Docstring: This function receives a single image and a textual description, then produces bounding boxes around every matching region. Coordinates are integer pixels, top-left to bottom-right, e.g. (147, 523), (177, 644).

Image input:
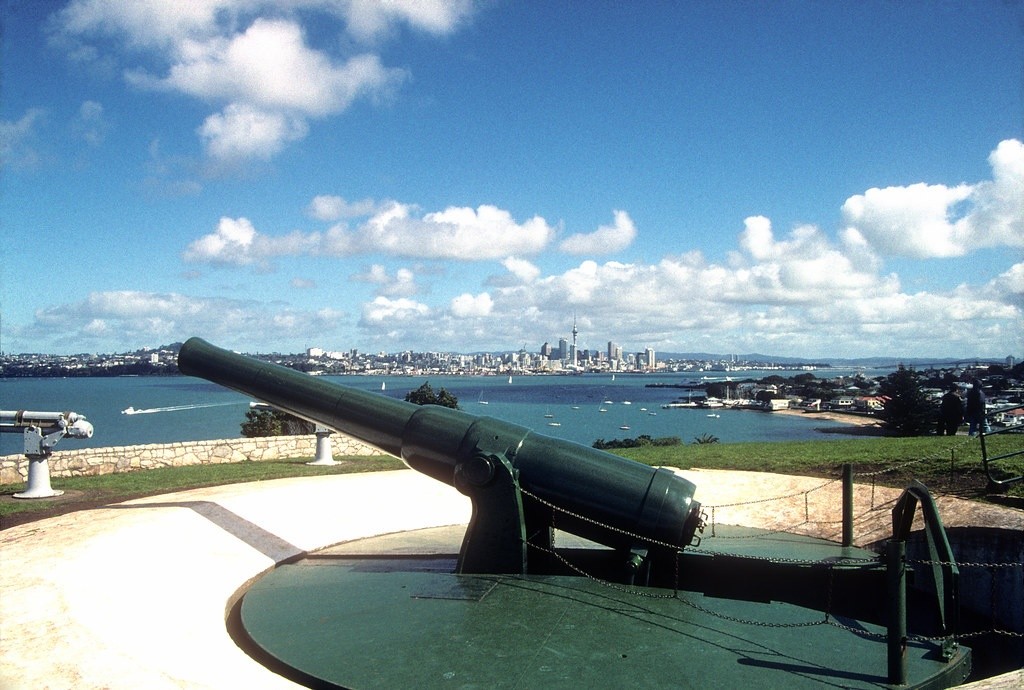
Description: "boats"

(693, 397), (724, 408)
(805, 398), (826, 413)
(120, 407), (135, 415)
